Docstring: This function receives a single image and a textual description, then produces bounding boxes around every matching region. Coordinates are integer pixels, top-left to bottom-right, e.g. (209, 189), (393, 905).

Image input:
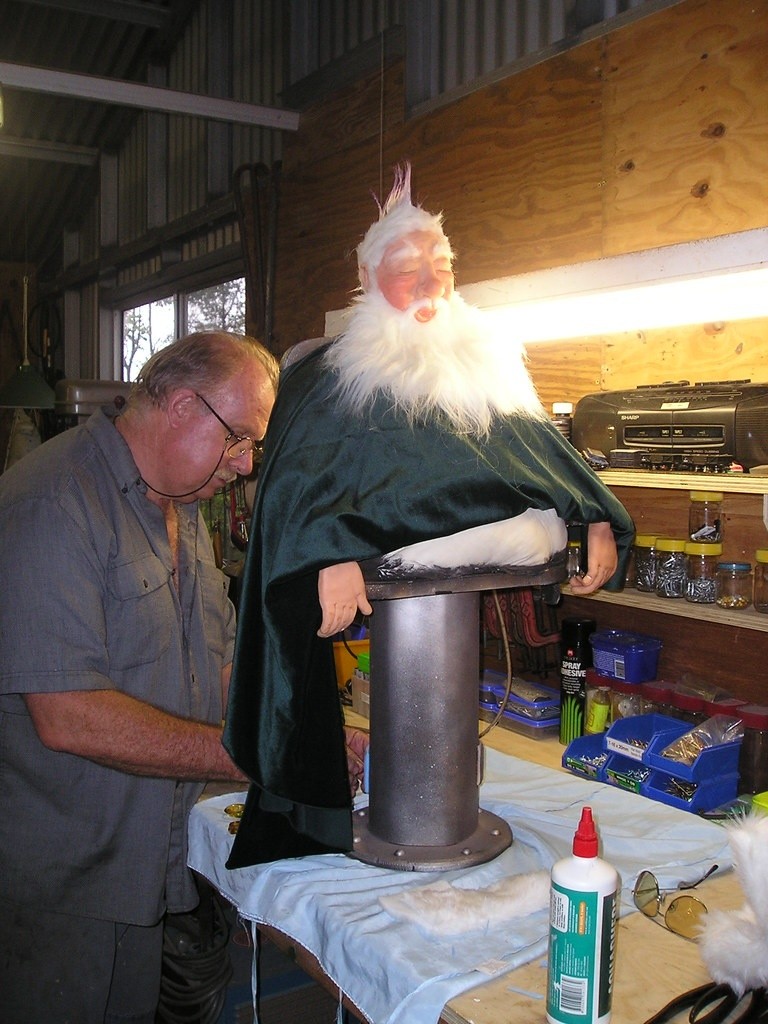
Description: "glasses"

(630, 865), (719, 945)
(195, 393), (264, 463)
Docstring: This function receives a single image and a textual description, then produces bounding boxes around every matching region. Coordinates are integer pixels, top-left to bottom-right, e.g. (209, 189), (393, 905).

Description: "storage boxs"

(333, 628), (743, 815)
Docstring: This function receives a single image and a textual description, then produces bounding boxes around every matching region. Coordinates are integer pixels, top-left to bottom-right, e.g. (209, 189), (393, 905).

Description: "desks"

(186, 687), (768, 1024)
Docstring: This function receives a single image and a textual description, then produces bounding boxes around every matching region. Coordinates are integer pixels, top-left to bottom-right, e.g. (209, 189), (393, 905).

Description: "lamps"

(0, 276), (57, 411)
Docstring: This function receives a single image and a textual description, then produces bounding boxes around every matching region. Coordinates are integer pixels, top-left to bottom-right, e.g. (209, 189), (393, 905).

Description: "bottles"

(559, 621), (767, 795)
(558, 490), (768, 615)
(548, 402), (573, 446)
(546, 806), (618, 1023)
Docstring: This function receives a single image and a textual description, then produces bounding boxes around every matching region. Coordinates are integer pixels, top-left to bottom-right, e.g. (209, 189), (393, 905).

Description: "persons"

(0, 330), (368, 1023)
(218, 160), (636, 868)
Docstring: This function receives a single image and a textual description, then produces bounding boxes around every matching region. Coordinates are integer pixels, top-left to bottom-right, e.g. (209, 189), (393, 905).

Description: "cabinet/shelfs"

(554, 467), (768, 633)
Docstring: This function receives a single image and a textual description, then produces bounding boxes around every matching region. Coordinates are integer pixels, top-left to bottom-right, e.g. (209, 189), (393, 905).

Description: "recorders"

(572, 378), (768, 473)
